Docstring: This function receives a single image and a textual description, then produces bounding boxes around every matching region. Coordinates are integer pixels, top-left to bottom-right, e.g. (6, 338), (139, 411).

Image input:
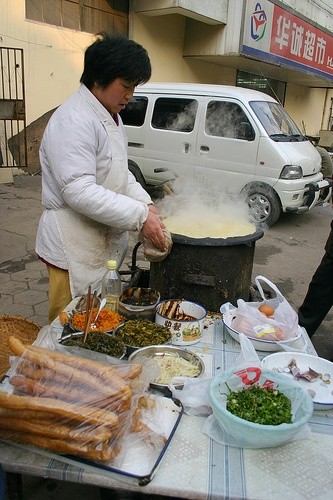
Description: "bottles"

(101, 259), (122, 314)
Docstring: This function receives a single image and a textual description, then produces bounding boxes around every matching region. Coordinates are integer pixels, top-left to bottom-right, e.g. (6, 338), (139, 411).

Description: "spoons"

(90, 299), (107, 330)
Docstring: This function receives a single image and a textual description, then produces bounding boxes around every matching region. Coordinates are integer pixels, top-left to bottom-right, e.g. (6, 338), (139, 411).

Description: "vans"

(118, 81), (333, 230)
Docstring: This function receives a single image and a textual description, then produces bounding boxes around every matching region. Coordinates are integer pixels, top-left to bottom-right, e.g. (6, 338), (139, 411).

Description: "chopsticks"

(81, 286), (97, 343)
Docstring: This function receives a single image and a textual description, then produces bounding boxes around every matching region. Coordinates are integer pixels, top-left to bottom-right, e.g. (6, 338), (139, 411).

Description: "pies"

(161, 215), (257, 239)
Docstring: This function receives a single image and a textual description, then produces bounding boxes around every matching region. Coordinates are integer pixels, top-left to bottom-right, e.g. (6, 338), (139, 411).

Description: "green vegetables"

(117, 318), (171, 347)
(222, 382), (293, 426)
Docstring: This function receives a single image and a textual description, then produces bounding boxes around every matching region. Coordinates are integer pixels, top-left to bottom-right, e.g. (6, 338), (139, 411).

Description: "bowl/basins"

(57, 331), (127, 360)
(117, 286), (160, 321)
(112, 322), (173, 357)
(155, 300), (207, 346)
(128, 345), (206, 396)
(221, 308), (301, 352)
(261, 352), (333, 411)
(67, 309), (127, 333)
(208, 366), (314, 448)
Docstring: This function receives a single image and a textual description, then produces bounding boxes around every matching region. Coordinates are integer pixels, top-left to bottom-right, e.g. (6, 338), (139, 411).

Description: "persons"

(312, 140), (333, 201)
(34, 31), (165, 326)
(298, 220), (333, 338)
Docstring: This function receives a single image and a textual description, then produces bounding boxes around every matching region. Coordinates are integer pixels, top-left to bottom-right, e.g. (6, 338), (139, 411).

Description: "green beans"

(58, 330), (127, 358)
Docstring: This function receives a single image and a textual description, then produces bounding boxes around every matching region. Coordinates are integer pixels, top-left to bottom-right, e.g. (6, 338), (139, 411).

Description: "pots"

(160, 210), (264, 245)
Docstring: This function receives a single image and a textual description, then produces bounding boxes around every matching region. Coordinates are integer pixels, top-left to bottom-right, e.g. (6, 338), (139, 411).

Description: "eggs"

(238, 305), (283, 341)
